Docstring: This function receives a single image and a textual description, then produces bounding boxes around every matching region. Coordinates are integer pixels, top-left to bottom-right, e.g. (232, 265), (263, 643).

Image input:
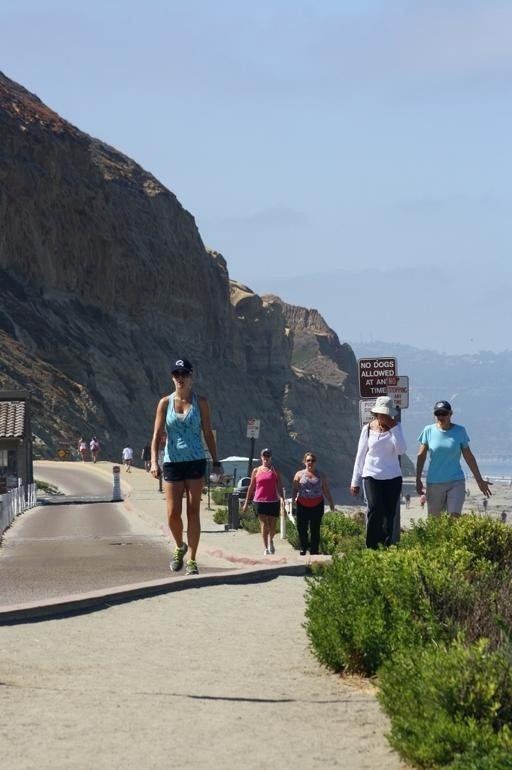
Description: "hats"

(261, 448), (272, 456)
(171, 359), (192, 373)
(434, 401), (451, 413)
(370, 396), (399, 416)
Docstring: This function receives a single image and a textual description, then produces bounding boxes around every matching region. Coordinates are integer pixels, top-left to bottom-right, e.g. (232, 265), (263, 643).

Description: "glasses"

(435, 411), (448, 416)
(174, 371), (188, 378)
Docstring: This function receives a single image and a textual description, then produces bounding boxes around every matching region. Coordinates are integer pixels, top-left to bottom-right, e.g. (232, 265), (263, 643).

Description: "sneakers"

(170, 543), (187, 571)
(185, 560), (199, 575)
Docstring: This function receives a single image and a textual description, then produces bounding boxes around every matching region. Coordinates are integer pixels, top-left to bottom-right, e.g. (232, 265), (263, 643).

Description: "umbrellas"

(210, 455), (259, 486)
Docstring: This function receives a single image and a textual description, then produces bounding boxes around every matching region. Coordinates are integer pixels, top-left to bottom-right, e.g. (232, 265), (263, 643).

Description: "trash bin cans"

(233, 477), (255, 529)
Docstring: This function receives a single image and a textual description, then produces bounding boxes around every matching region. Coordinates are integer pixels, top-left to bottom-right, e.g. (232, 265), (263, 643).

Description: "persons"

(420, 492), (425, 508)
(416, 401), (494, 520)
(481, 498), (488, 512)
(292, 453), (336, 555)
(244, 448), (286, 555)
(349, 396), (407, 551)
(122, 444), (133, 473)
(466, 488), (469, 497)
(90, 435), (101, 463)
(151, 358), (223, 577)
(141, 442), (150, 472)
(404, 493), (410, 507)
(79, 436), (88, 462)
(500, 511), (507, 522)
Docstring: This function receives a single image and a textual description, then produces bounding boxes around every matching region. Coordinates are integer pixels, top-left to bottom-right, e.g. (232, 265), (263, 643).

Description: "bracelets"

(246, 498), (250, 500)
(212, 462), (221, 467)
